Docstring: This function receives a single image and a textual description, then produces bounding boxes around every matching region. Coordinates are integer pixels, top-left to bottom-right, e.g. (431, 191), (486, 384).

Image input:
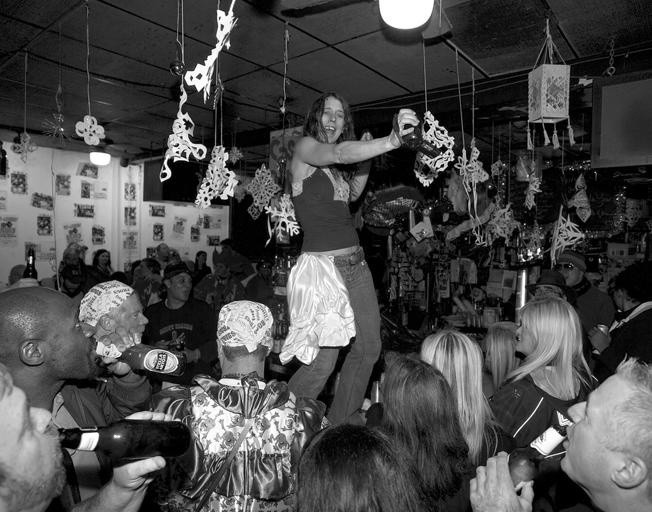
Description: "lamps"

(376, 1), (435, 31)
(87, 142), (113, 168)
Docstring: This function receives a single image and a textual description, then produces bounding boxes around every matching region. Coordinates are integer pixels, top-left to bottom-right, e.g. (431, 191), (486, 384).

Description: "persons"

(278, 91), (420, 427)
(0, 241), (652, 512)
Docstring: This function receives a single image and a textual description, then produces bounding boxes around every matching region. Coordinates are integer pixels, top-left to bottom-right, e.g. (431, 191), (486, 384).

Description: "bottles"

(24, 247), (38, 277)
(402, 127), (421, 149)
(56, 418), (192, 459)
(270, 254), (299, 361)
(91, 339), (187, 378)
(508, 423), (565, 496)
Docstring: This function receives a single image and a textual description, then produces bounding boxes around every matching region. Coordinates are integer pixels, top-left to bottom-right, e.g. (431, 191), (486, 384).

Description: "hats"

(524, 271), (570, 297)
(257, 258), (274, 272)
(160, 261), (192, 290)
(557, 249), (588, 273)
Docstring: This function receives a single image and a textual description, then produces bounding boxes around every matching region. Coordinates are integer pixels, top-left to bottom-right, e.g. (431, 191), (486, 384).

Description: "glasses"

(554, 264), (574, 271)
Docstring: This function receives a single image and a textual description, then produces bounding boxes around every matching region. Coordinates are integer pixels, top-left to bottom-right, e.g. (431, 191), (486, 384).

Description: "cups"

(371, 381), (384, 404)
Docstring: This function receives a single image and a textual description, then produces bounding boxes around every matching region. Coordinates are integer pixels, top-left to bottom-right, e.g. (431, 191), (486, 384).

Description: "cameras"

(402, 114), (440, 159)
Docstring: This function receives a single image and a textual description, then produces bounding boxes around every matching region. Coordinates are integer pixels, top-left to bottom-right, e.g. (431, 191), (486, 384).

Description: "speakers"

(592, 70), (652, 168)
(229, 193), (268, 263)
(143, 160), (230, 206)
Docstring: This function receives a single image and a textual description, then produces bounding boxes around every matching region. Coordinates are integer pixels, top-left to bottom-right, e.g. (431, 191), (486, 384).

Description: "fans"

(278, 1), (468, 41)
